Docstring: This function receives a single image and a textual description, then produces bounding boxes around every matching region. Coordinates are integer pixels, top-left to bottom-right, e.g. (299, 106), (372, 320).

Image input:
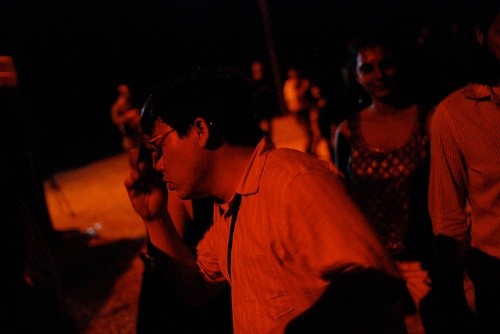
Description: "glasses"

(141, 118), (213, 156)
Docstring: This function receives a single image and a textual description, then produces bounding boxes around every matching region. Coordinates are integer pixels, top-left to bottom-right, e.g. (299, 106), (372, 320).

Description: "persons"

(334, 32), (474, 334)
(425, 0), (500, 334)
(110, 60), (351, 175)
(123, 66), (419, 334)
